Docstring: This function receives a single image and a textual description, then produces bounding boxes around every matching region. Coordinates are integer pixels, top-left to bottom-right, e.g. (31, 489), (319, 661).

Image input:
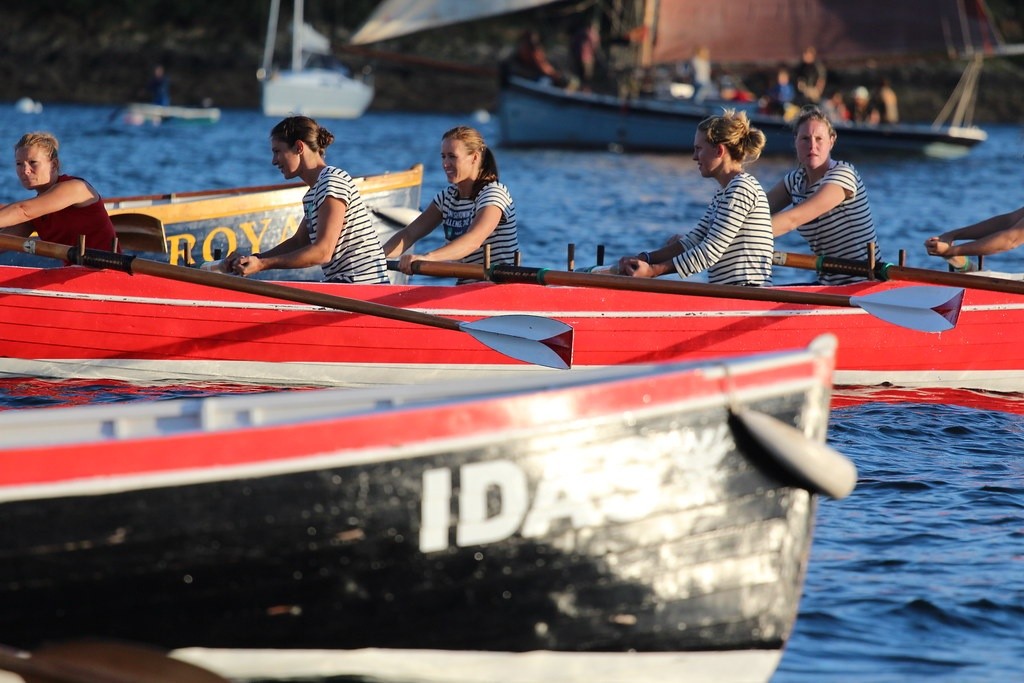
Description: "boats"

(103, 164), (427, 284)
(0, 262), (1023, 414)
(0, 332), (859, 683)
(111, 102), (220, 128)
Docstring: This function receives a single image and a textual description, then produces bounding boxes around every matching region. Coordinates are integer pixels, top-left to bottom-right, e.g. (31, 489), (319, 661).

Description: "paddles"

(386, 256), (967, 333)
(0, 227), (575, 371)
(770, 247), (1024, 298)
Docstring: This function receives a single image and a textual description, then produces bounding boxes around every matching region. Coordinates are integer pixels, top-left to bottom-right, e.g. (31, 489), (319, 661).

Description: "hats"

(849, 85), (869, 99)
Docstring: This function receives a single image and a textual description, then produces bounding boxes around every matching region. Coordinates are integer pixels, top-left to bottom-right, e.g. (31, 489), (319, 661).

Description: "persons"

(767, 109), (884, 284)
(382, 125), (519, 284)
(0, 132), (125, 267)
(925, 206), (1024, 259)
(529, 37), (905, 120)
(619, 110), (772, 286)
(233, 115), (389, 284)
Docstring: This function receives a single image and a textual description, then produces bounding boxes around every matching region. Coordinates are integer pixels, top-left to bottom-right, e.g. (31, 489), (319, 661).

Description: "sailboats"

(493, 0), (991, 161)
(253, 0), (376, 118)
(330, 1), (560, 113)
